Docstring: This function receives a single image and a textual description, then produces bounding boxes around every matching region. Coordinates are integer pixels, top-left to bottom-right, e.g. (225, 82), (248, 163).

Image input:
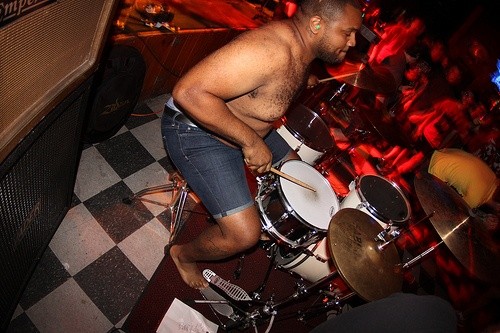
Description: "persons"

(302, 0), (500, 333)
(161, 0), (366, 289)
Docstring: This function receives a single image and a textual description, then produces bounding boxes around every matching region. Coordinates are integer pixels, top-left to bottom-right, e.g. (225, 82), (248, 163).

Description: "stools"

(121, 164), (201, 257)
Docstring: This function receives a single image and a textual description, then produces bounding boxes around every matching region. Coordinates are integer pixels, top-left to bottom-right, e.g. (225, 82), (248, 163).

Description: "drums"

(277, 100), (333, 166)
(276, 228), (338, 281)
(334, 172), (412, 238)
(255, 158), (340, 248)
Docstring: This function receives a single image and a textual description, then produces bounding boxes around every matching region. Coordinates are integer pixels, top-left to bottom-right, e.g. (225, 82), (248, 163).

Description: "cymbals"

(328, 207), (406, 302)
(360, 85), (415, 147)
(412, 169), (500, 287)
(324, 52), (399, 93)
